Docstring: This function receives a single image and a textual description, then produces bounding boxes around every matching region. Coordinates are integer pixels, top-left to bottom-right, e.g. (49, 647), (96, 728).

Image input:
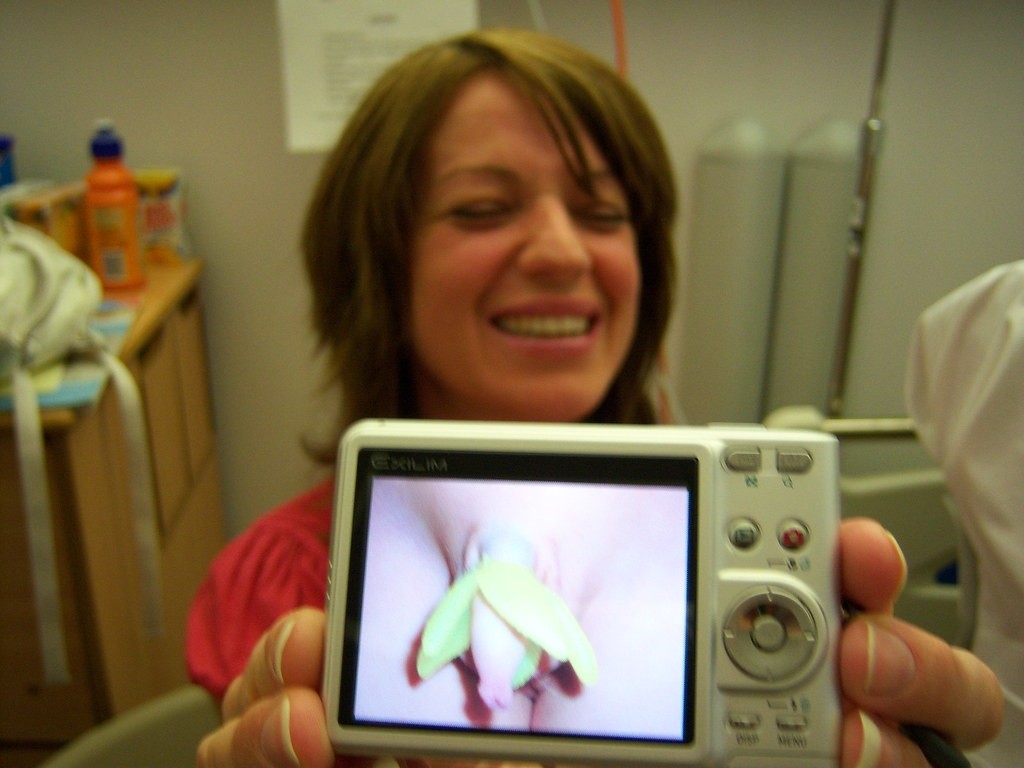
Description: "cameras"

(321, 419), (840, 768)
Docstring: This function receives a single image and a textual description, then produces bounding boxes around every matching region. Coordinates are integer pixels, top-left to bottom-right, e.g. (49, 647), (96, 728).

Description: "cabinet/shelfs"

(1, 257), (226, 768)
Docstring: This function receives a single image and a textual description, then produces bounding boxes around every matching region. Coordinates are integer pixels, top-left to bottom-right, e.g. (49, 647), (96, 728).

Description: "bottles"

(87, 124), (145, 291)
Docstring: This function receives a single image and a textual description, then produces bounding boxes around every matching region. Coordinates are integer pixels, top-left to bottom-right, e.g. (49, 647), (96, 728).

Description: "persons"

(182, 28), (1007, 768)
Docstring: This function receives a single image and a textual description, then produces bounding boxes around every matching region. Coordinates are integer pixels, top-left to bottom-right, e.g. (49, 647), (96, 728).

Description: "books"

(0, 299), (134, 410)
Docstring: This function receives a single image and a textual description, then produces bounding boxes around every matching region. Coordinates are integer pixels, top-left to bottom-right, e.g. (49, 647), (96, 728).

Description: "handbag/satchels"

(0, 217), (102, 373)
